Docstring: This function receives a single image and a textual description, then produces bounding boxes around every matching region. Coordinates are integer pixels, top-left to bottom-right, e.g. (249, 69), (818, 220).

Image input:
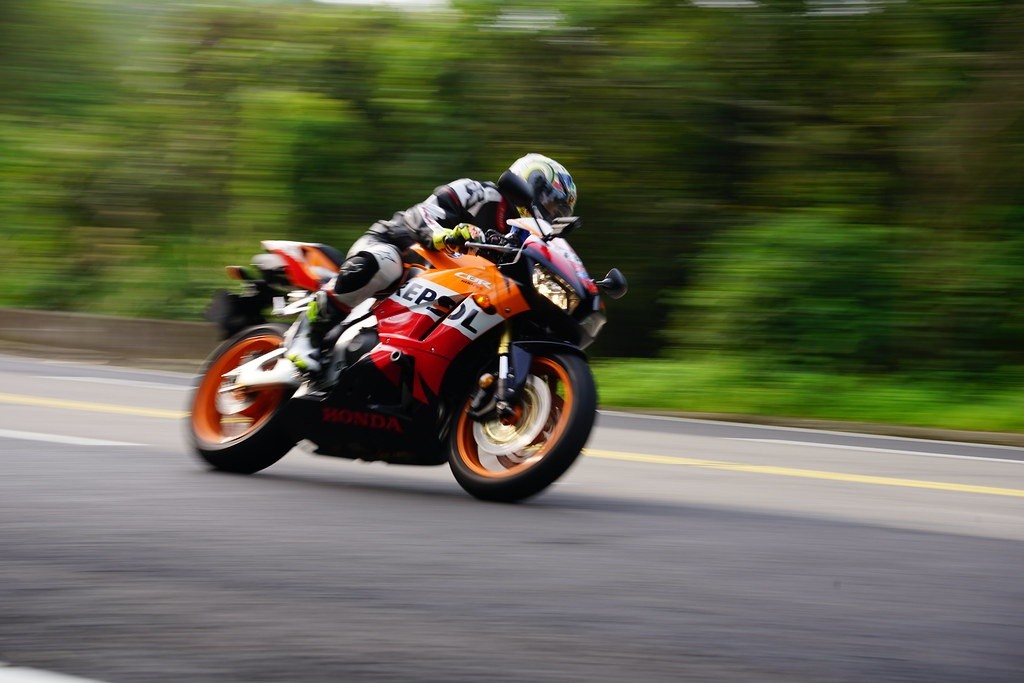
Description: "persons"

(283, 153), (577, 372)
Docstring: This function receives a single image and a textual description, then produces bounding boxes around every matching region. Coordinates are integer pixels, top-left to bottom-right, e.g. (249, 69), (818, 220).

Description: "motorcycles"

(188, 167), (628, 501)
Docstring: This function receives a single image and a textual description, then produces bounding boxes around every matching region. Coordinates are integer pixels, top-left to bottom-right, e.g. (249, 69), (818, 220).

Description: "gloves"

(434, 223), (486, 255)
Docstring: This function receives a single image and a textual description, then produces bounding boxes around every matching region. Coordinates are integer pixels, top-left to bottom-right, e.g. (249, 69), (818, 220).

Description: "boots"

(280, 289), (352, 371)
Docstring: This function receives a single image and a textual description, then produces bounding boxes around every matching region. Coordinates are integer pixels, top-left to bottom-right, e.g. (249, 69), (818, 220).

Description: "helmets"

(498, 152), (577, 223)
